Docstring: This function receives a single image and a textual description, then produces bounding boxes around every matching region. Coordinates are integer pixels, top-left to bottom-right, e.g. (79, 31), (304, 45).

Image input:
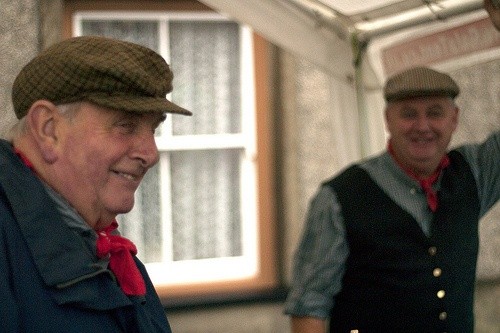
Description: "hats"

(382, 65), (460, 100)
(10, 34), (195, 117)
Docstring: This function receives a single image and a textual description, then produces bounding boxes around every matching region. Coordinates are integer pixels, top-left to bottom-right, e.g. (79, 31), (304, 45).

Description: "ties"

(387, 136), (449, 212)
(9, 144), (147, 296)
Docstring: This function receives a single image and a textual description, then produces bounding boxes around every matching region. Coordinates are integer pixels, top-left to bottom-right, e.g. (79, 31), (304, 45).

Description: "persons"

(283, 67), (500, 333)
(0, 37), (193, 333)
